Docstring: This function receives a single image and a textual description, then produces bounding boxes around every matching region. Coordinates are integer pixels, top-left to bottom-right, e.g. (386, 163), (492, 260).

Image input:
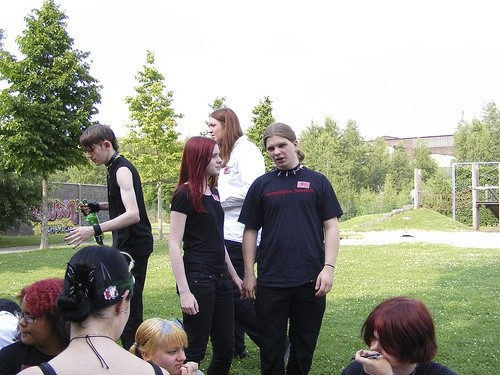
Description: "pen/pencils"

(352, 354), (382, 360)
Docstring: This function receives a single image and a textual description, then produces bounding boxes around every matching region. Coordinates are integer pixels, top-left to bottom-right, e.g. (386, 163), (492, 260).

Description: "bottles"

(82, 199), (104, 244)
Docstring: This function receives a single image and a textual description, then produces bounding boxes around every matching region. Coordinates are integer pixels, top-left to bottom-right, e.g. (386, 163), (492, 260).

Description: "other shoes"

(283, 335), (290, 362)
(233, 349), (247, 358)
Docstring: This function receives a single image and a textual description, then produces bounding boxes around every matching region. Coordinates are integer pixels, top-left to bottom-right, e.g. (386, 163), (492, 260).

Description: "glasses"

(141, 317), (184, 346)
(14, 308), (52, 323)
(120, 251), (135, 274)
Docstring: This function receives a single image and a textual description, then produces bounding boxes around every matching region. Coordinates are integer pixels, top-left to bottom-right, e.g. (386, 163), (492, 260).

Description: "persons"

(64, 124), (153, 350)
(340, 296), (457, 375)
(208, 108), (291, 363)
(168, 136), (256, 375)
(238, 123), (343, 375)
(0, 245), (205, 375)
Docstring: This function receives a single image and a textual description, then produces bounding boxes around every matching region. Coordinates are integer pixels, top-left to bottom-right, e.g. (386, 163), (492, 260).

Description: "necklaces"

(276, 163), (304, 177)
(105, 151), (120, 166)
(412, 363), (418, 375)
(69, 333), (116, 369)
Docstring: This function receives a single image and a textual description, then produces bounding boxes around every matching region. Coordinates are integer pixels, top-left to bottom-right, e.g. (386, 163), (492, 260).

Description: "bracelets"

(92, 223), (102, 237)
(324, 263), (335, 268)
